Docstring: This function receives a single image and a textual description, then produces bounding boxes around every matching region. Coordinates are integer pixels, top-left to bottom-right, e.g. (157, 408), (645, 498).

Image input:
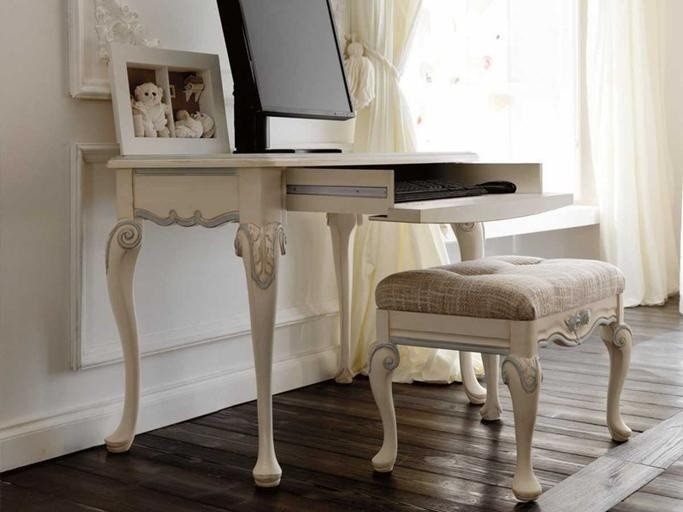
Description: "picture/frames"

(107, 41), (231, 157)
(66, 1), (234, 109)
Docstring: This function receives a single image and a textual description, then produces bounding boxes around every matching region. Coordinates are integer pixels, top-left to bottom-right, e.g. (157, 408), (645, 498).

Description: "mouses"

(479, 181), (516, 193)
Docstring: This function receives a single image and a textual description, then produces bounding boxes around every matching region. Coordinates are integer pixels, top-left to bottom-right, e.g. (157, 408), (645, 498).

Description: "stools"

(365, 254), (634, 500)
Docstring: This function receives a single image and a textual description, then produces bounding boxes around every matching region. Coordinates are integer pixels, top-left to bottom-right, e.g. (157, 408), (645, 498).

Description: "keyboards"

(395, 179), (488, 203)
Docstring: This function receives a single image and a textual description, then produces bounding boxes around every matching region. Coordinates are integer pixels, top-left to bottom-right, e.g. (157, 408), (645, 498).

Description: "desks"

(103, 150), (573, 486)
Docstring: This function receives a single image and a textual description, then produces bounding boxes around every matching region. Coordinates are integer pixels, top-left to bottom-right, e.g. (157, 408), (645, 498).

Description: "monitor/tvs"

(216, 0), (355, 153)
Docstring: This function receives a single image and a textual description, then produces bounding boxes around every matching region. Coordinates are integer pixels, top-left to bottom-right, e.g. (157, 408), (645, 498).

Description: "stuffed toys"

(134, 82), (170, 137)
(174, 110), (215, 138)
